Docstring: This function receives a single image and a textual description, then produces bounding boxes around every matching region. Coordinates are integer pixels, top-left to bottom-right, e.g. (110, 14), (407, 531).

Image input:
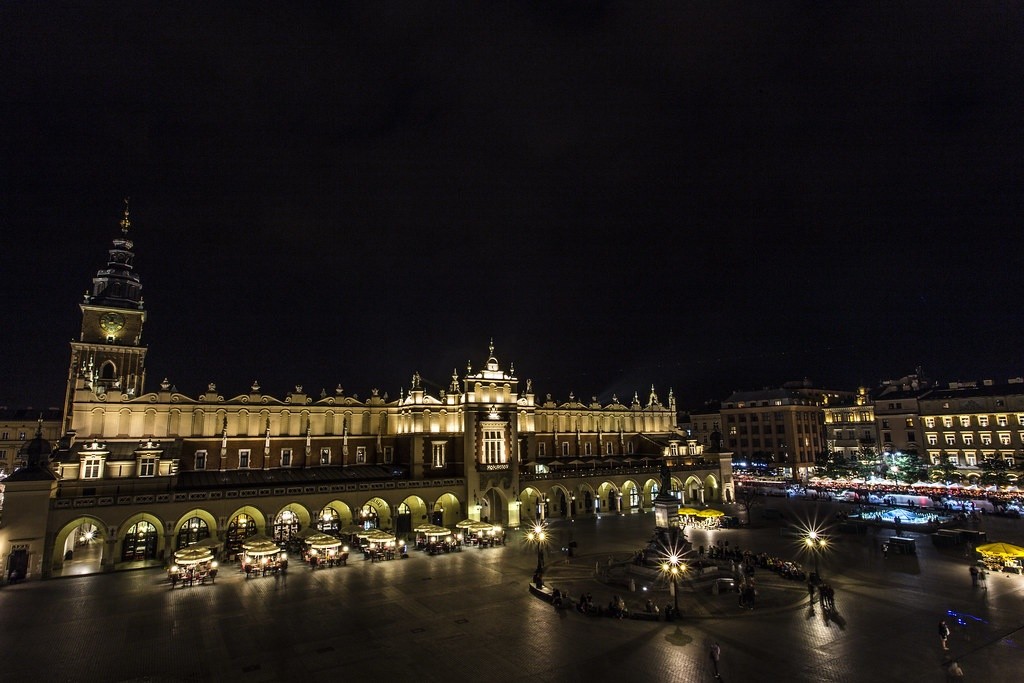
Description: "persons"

(709, 641), (721, 678)
(938, 618), (951, 651)
(969, 565), (989, 589)
(732, 563), (760, 613)
(775, 561), (821, 586)
(881, 542), (890, 561)
(948, 661), (963, 683)
(696, 539), (778, 565)
(646, 598), (684, 617)
(806, 582), (835, 609)
(907, 499), (915, 506)
(551, 588), (628, 622)
(894, 516), (902, 528)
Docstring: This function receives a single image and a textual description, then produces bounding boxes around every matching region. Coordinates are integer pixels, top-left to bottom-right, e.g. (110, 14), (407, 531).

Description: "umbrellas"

(174, 538), (225, 564)
(242, 533), (280, 563)
(294, 526), (342, 563)
(975, 543), (1024, 559)
(414, 523), (451, 541)
(677, 507), (725, 524)
(456, 518), (493, 537)
(339, 524), (396, 549)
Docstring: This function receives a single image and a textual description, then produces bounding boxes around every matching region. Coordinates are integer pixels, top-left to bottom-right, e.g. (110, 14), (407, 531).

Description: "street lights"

(806, 530), (828, 575)
(527, 524), (549, 566)
(661, 561), (686, 609)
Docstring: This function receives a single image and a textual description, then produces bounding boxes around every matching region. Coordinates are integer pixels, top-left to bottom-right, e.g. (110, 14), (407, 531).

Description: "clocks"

(99, 312), (125, 333)
